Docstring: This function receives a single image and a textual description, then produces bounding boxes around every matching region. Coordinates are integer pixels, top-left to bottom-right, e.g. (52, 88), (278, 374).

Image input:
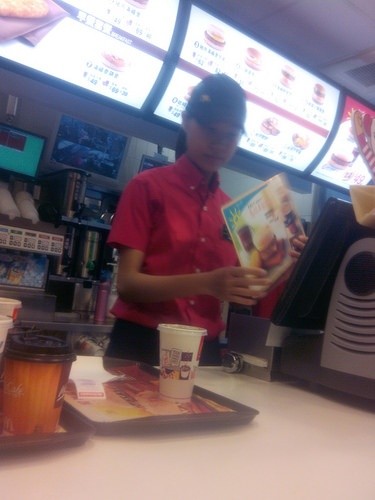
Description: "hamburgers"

(312, 83), (325, 105)
(245, 48), (261, 69)
(329, 148), (354, 169)
(280, 65), (296, 87)
(253, 225), (278, 261)
(203, 29), (225, 50)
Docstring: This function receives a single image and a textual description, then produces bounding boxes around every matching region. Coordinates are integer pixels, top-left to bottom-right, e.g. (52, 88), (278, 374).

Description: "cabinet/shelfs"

(42, 215), (113, 285)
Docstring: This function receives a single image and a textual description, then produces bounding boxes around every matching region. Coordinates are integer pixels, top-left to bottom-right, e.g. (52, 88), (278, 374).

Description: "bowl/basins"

(349, 184), (375, 227)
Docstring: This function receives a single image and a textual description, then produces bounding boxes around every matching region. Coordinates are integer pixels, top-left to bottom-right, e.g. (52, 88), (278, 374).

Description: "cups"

(236, 202), (298, 259)
(156, 323), (207, 398)
(0, 296), (77, 436)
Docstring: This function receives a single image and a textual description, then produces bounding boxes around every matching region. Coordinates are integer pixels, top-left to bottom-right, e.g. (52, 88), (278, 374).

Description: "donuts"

(103, 53), (127, 71)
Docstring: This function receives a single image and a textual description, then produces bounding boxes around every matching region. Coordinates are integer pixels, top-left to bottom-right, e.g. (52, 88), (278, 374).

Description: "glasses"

(197, 121), (244, 141)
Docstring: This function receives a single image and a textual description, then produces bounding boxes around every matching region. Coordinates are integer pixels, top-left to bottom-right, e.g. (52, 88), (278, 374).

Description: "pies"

(0, 0), (48, 18)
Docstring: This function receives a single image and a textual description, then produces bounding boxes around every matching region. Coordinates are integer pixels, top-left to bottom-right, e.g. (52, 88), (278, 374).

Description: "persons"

(107, 74), (312, 366)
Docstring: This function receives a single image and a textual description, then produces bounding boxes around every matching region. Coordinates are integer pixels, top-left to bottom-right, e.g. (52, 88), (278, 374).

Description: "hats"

(186, 74), (246, 121)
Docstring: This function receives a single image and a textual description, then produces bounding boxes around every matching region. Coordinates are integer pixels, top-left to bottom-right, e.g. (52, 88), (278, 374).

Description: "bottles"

(94, 278), (109, 324)
